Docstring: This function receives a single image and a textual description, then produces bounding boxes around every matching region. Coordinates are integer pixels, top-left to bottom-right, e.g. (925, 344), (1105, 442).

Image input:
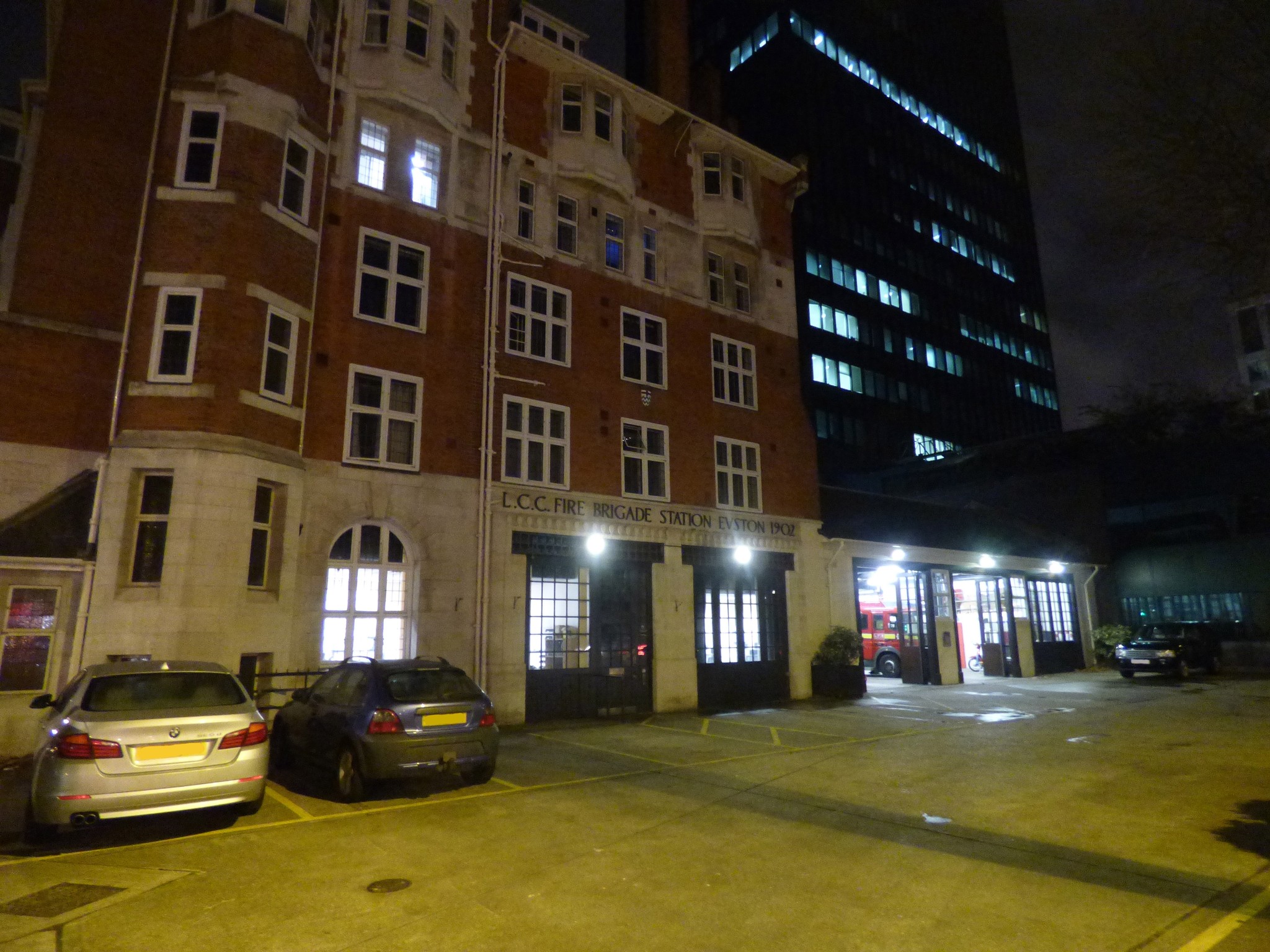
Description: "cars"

(30, 660), (270, 838)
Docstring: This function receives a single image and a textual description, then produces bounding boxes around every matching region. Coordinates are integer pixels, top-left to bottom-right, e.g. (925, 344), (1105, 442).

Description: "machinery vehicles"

(856, 599), (921, 680)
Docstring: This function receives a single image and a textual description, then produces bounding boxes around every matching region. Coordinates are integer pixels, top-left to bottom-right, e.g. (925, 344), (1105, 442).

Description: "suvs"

(1115, 620), (1212, 682)
(278, 652), (502, 797)
